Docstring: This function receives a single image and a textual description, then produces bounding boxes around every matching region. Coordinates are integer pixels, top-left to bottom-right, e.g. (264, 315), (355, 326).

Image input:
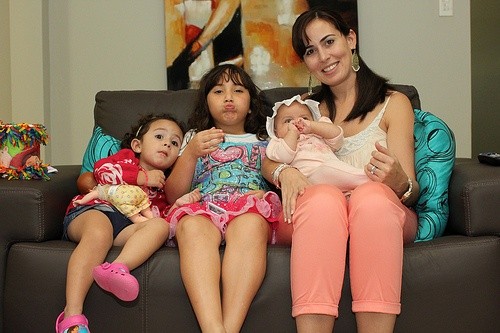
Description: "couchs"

(0, 85), (500, 333)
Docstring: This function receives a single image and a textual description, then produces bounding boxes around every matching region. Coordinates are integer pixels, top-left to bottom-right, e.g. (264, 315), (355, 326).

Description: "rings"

(370, 165), (376, 175)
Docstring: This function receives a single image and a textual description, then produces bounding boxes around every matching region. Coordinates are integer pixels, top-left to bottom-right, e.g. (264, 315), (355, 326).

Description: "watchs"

(399, 178), (414, 204)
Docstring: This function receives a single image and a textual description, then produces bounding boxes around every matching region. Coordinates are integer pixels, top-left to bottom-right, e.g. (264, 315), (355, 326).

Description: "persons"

(73, 172), (154, 224)
(163, 63), (272, 333)
(63, 114), (182, 333)
(261, 6), (418, 333)
(265, 94), (370, 190)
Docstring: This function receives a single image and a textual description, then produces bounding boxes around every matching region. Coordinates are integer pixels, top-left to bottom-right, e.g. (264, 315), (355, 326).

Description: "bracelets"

(142, 170), (149, 187)
(272, 163), (297, 189)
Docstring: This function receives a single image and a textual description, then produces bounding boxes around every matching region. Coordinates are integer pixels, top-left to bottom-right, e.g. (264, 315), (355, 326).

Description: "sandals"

(92, 261), (140, 302)
(56, 310), (91, 333)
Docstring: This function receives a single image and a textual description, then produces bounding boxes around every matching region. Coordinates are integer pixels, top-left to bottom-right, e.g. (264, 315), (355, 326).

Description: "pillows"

(80, 126), (123, 176)
(409, 109), (456, 243)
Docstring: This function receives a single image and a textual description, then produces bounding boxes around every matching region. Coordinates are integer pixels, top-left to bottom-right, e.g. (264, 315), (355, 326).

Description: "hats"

(265, 94), (322, 140)
